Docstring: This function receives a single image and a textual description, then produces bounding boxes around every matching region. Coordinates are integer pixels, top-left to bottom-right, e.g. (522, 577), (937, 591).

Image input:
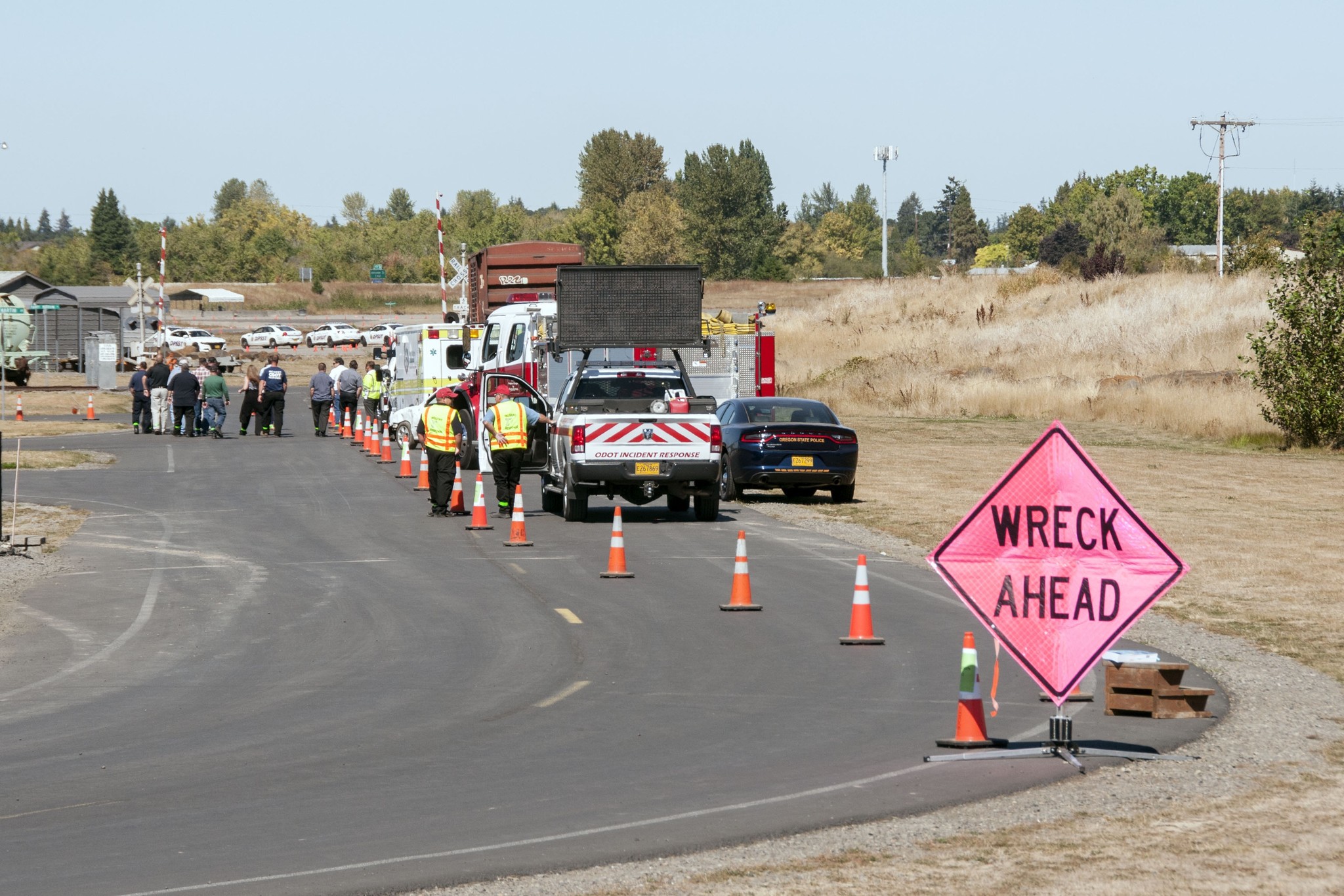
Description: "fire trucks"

(443, 293), (778, 469)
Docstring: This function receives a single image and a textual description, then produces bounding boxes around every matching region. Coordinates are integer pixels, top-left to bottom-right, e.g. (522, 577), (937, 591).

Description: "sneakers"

(434, 512), (453, 518)
(491, 512), (510, 518)
(427, 512), (435, 516)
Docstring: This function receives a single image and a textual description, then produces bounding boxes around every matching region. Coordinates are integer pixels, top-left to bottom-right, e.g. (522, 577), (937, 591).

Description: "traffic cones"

(465, 472), (494, 530)
(1039, 682), (1093, 700)
(449, 461), (471, 514)
(938, 633), (1009, 748)
(243, 343), (387, 352)
(838, 553), (886, 644)
(413, 448), (430, 491)
(14, 394), (101, 421)
(171, 313), (427, 334)
(327, 403), (397, 464)
(599, 507), (635, 577)
(394, 435), (418, 478)
(502, 485), (534, 546)
(719, 530), (763, 611)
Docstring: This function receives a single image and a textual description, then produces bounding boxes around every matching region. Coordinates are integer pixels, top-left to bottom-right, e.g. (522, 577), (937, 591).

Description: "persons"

(337, 360), (363, 435)
(329, 357), (349, 433)
(191, 357), (211, 436)
(362, 361), (381, 430)
(416, 388), (464, 518)
(206, 357), (223, 376)
(201, 365), (230, 439)
(238, 365), (261, 435)
(167, 363), (200, 437)
(142, 354), (170, 435)
(257, 355), (287, 437)
(309, 363), (335, 437)
(167, 359), (190, 436)
(129, 361), (152, 434)
(260, 355), (272, 393)
(481, 384), (552, 518)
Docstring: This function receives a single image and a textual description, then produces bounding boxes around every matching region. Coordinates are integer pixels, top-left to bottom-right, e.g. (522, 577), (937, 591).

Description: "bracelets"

(258, 394), (262, 396)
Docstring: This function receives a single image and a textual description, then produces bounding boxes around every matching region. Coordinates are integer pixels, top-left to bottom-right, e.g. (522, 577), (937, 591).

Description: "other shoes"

(202, 430), (208, 436)
(170, 430), (181, 437)
(212, 434), (216, 439)
(214, 426), (223, 438)
(269, 427), (275, 430)
(274, 432), (280, 437)
(239, 432), (246, 435)
(314, 430), (320, 436)
(186, 432), (194, 437)
(255, 434), (259, 435)
(320, 433), (328, 437)
(260, 431), (268, 437)
(134, 424), (164, 434)
(194, 430), (201, 436)
(328, 429), (339, 434)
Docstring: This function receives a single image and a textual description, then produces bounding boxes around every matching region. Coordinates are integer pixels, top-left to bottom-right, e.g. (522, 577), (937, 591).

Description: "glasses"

(333, 362), (337, 365)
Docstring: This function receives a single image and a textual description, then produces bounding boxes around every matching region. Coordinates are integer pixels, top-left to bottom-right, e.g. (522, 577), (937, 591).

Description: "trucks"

(468, 241), (585, 324)
(0, 292), (51, 387)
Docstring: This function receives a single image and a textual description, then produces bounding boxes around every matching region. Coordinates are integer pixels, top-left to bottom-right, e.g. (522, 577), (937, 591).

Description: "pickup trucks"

(476, 366), (725, 521)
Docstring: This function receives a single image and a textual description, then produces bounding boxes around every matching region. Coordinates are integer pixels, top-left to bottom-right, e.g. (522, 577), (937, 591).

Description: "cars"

(240, 324), (303, 349)
(153, 326), (227, 353)
(392, 385), (458, 449)
(359, 323), (405, 347)
(714, 397), (860, 504)
(305, 323), (361, 348)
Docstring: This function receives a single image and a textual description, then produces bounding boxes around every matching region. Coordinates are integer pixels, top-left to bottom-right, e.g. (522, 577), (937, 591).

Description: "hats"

(179, 359), (188, 365)
(208, 357), (216, 363)
(490, 384), (510, 395)
(436, 388), (458, 398)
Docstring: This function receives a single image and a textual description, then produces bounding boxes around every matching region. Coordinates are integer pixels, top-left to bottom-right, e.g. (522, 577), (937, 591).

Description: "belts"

(155, 386), (167, 388)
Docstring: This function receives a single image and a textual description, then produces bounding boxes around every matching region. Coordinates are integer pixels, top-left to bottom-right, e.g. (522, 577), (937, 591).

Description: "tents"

(169, 288), (245, 310)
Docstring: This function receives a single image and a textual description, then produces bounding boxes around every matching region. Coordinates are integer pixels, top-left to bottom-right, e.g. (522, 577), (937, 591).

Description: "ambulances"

(371, 322), (488, 442)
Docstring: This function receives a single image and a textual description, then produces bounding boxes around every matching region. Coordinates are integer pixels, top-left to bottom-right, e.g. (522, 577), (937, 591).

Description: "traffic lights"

(125, 316), (138, 331)
(146, 315), (159, 330)
(444, 311), (459, 323)
